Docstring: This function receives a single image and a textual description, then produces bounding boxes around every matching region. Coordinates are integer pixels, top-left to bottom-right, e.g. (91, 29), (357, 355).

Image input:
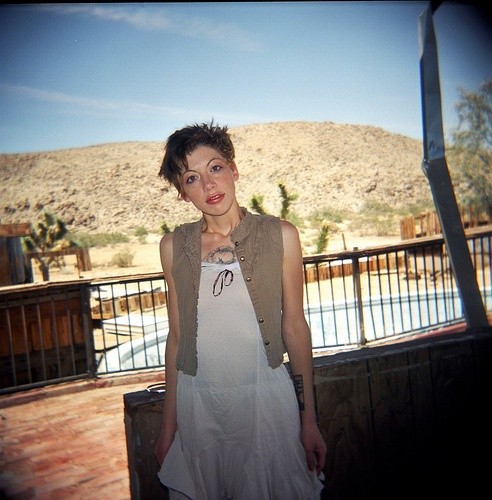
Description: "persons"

(152, 123), (328, 500)
